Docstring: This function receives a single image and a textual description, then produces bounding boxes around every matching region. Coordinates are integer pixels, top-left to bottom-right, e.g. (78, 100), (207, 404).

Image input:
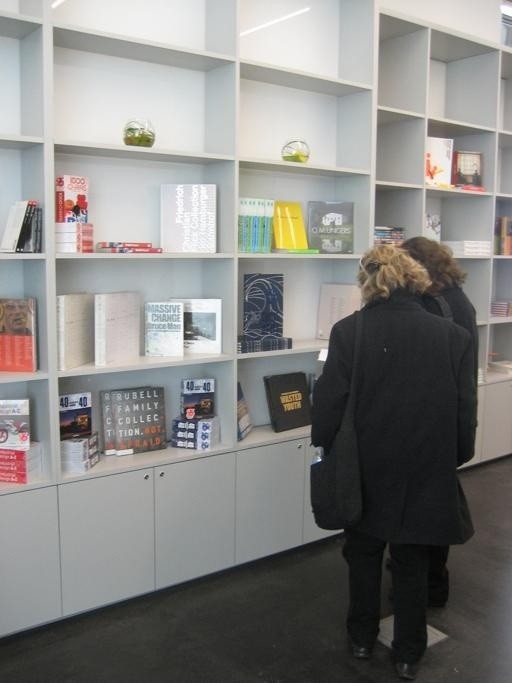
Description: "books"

(263, 371), (312, 433)
(58, 392), (100, 474)
(316, 283), (361, 339)
(374, 133), (511, 384)
(1, 297), (38, 372)
(1, 399), (42, 484)
(237, 381), (252, 440)
(2, 200), (42, 253)
(100, 387), (167, 456)
(160, 184), (217, 254)
(238, 196), (355, 254)
(171, 378), (220, 450)
(236, 274), (293, 354)
(56, 291), (222, 370)
(95, 242), (163, 253)
(54, 175), (94, 253)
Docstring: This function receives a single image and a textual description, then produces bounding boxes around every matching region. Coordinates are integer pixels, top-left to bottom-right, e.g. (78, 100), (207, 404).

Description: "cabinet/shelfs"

(0, 0), (350, 638)
(340, 0), (509, 475)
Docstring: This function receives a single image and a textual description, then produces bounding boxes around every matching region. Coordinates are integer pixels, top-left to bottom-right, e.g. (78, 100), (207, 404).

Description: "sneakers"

(386, 586), (446, 608)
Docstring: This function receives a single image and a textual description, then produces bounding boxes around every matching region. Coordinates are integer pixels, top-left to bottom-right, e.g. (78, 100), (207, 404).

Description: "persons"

(384, 235), (475, 609)
(309, 237), (477, 680)
(1, 298), (33, 336)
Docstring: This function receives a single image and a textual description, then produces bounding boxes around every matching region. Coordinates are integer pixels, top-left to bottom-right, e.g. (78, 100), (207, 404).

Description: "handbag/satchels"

(310, 461), (343, 529)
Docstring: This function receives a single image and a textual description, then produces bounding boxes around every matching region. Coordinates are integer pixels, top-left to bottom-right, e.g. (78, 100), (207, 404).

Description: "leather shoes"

(395, 662), (419, 680)
(347, 634), (372, 658)
